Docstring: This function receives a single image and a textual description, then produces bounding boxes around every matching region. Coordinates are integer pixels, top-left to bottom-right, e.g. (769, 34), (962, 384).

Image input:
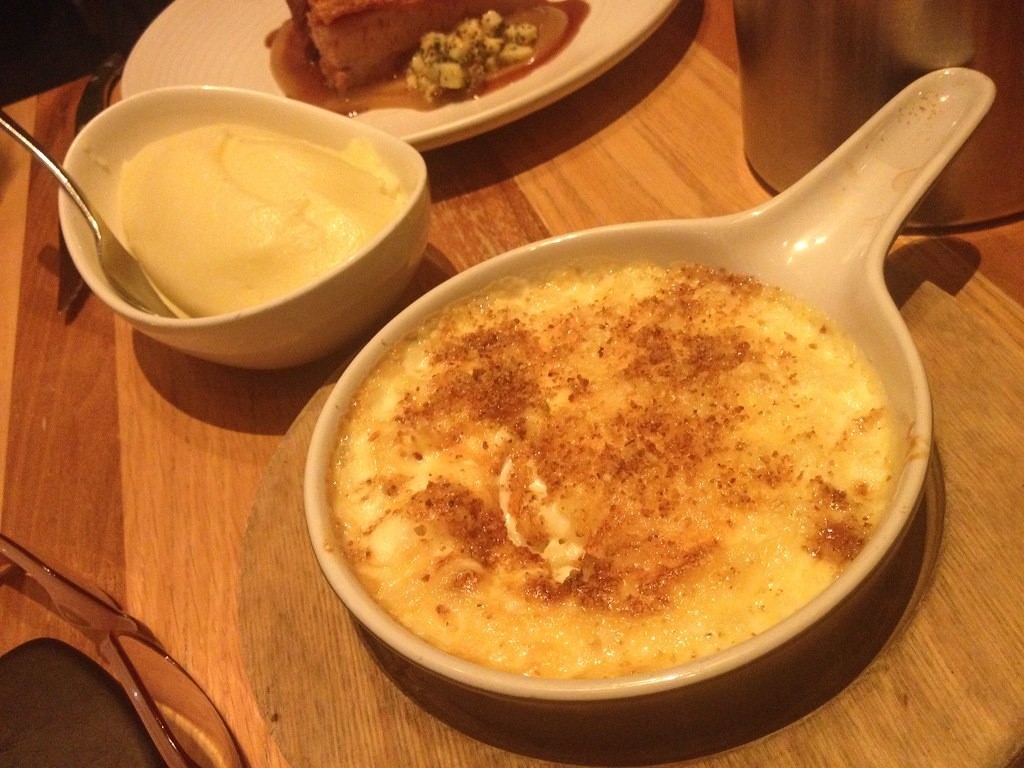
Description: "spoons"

(0, 107), (180, 320)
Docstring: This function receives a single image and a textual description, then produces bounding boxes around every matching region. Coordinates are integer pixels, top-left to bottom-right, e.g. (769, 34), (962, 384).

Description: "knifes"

(56, 50), (125, 316)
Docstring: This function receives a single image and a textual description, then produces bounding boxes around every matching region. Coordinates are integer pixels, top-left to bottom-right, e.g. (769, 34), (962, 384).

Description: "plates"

(300, 65), (996, 718)
(119, 1), (679, 152)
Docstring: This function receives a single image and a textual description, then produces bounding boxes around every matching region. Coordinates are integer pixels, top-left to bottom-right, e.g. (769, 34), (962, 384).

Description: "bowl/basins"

(55, 87), (432, 369)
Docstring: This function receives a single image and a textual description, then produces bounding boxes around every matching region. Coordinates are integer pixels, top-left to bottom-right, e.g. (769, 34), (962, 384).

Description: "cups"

(730, 0), (1024, 235)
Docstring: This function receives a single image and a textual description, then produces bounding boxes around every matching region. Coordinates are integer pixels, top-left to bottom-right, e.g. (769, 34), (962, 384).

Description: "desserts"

(287, 0), (549, 94)
